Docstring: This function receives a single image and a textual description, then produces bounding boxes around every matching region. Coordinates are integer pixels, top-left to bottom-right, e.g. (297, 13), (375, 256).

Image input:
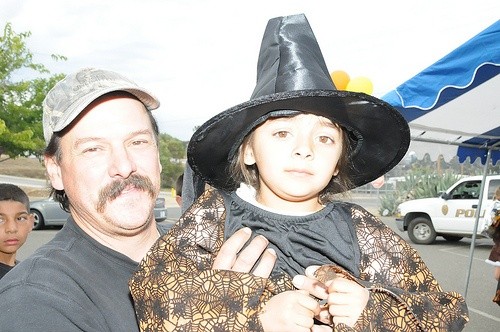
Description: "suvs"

(395, 175), (500, 245)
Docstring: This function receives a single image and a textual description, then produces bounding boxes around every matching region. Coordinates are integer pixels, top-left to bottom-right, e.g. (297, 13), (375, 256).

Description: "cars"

(29, 188), (168, 231)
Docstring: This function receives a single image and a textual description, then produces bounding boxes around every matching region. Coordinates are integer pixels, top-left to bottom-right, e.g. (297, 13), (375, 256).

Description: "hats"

(42, 67), (160, 148)
(187, 13), (411, 195)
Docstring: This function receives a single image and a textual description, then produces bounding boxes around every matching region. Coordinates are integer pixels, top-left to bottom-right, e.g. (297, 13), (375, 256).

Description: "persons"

(0, 183), (34, 281)
(1, 65), (175, 332)
(485, 183), (500, 305)
(129, 13), (469, 332)
